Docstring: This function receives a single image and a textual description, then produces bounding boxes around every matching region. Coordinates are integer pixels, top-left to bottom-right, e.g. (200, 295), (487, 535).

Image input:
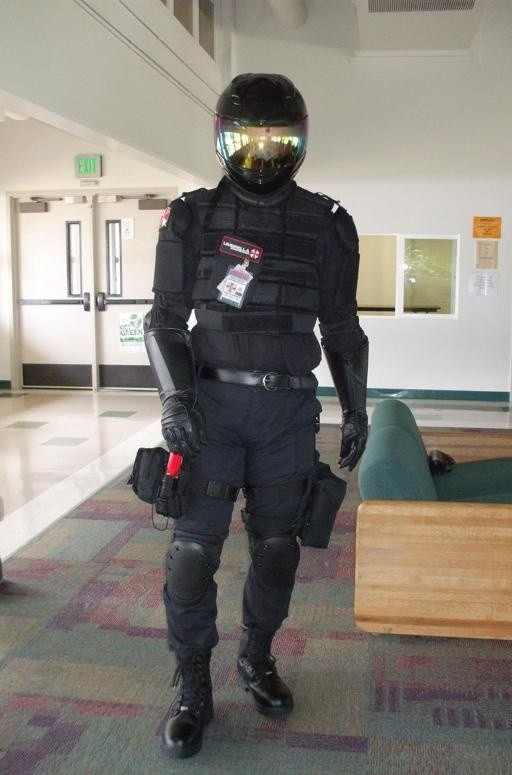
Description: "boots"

(162, 649), (214, 758)
(236, 620), (294, 717)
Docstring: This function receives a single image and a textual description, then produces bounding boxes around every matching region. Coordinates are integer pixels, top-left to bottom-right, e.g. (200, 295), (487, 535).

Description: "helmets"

(214, 73), (308, 206)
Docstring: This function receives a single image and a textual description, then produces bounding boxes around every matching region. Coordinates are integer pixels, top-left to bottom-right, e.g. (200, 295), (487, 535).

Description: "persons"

(143, 74), (369, 759)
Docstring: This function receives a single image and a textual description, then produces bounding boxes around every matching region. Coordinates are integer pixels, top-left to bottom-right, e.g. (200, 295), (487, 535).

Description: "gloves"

(159, 396), (207, 459)
(337, 412), (368, 472)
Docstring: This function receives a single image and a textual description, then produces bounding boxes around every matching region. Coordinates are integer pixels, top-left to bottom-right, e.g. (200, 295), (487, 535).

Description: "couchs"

(354, 398), (512, 643)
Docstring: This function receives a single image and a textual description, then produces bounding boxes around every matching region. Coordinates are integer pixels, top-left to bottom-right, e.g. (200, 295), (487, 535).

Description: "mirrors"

(402, 238), (456, 315)
(355, 234), (397, 316)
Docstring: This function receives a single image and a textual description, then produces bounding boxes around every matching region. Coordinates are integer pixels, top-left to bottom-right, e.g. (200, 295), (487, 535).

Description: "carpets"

(0, 421), (511, 775)
